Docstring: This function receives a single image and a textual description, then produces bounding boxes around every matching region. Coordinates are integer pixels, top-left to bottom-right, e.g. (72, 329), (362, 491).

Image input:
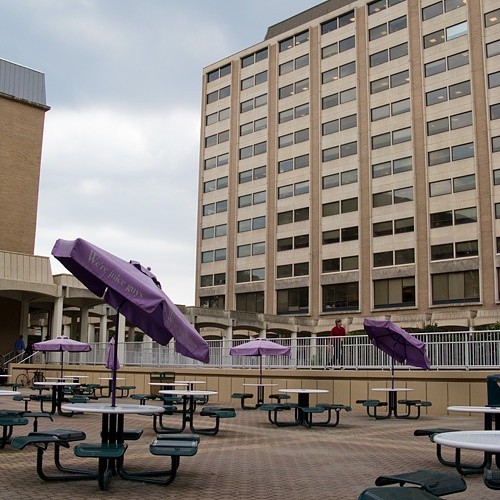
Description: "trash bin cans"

(146, 370), (177, 398)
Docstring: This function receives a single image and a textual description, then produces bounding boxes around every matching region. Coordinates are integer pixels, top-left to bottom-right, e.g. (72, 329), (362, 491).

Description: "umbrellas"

(363, 317), (431, 389)
(229, 339), (293, 384)
(52, 239), (210, 407)
(105, 337), (120, 377)
(32, 336), (92, 378)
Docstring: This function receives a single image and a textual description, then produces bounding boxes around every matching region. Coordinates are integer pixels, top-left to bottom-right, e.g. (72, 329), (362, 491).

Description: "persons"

(15, 335), (26, 362)
(329, 318), (346, 365)
(26, 342), (34, 362)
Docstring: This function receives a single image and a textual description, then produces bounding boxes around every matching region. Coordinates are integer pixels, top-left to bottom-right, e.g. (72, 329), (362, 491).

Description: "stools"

(259, 394), (292, 424)
(361, 466), (468, 500)
(130, 393), (156, 406)
(0, 410), (53, 448)
(414, 427), (459, 464)
(96, 426), (146, 439)
(399, 398), (433, 420)
(30, 395), (53, 412)
(150, 433), (201, 485)
(74, 441), (128, 485)
(117, 385), (137, 398)
(232, 393), (261, 410)
(199, 406), (237, 436)
(301, 400), (354, 429)
(355, 399), (392, 420)
(10, 427), (87, 482)
(62, 383), (99, 403)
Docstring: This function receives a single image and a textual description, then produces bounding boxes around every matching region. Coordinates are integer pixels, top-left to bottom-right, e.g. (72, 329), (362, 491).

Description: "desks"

(446, 402), (498, 414)
(59, 400), (165, 418)
(32, 381), (81, 386)
(148, 382), (206, 386)
(157, 389), (219, 397)
(96, 377), (127, 381)
(243, 382), (278, 387)
(63, 375), (90, 379)
(372, 387), (413, 392)
(0, 390), (22, 397)
(277, 387), (330, 394)
(433, 427), (500, 500)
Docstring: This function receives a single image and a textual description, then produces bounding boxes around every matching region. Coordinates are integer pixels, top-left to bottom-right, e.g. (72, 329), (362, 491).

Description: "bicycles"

(15, 369), (46, 388)
